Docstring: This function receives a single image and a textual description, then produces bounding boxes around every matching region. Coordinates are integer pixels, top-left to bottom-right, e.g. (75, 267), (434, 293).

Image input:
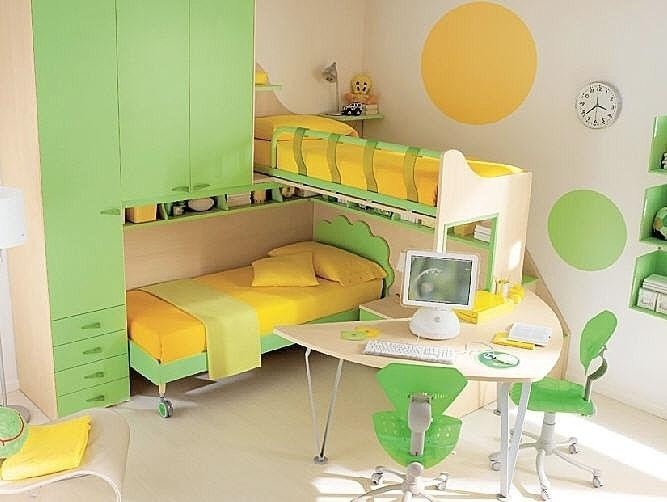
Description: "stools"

(0, 404), (130, 502)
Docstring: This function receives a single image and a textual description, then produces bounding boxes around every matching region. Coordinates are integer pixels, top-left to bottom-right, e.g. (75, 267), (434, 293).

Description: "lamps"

(326, 59), (342, 113)
(0, 183), (26, 251)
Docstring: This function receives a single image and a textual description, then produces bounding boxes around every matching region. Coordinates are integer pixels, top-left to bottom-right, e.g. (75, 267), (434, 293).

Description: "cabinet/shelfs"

(628, 114), (667, 318)
(116, 0), (256, 204)
(1, 1), (132, 419)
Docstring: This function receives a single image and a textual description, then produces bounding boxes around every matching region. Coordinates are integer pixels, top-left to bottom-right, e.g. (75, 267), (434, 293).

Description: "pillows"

(254, 114), (360, 146)
(248, 239), (390, 289)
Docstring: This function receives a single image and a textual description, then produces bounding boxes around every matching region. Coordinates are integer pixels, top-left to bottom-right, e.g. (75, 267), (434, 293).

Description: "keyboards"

(362, 340), (453, 364)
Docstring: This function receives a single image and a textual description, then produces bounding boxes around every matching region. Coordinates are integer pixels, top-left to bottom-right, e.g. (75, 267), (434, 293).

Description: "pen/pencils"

(492, 271), (512, 284)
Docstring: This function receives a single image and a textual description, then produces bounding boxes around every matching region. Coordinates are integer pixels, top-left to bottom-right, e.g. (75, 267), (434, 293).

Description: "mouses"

(497, 355), (519, 365)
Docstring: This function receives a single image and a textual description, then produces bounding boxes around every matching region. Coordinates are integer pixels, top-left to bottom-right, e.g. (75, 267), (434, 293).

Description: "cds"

(356, 325), (380, 338)
(341, 329), (364, 341)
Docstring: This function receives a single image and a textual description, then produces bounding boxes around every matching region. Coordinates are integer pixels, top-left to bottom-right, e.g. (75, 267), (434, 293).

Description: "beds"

(257, 110), (531, 242)
(120, 213), (396, 418)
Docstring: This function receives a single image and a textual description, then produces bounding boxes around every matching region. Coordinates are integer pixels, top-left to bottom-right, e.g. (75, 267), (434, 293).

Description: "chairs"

(488, 310), (617, 500)
(349, 363), (468, 502)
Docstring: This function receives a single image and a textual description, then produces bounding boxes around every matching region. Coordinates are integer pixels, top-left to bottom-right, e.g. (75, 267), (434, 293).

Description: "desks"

(259, 288), (564, 500)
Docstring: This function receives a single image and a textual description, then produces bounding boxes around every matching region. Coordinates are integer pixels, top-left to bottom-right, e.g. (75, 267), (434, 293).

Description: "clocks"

(574, 81), (622, 130)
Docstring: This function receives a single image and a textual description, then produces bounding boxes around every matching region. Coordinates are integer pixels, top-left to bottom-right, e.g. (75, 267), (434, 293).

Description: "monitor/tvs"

(401, 250), (477, 340)
